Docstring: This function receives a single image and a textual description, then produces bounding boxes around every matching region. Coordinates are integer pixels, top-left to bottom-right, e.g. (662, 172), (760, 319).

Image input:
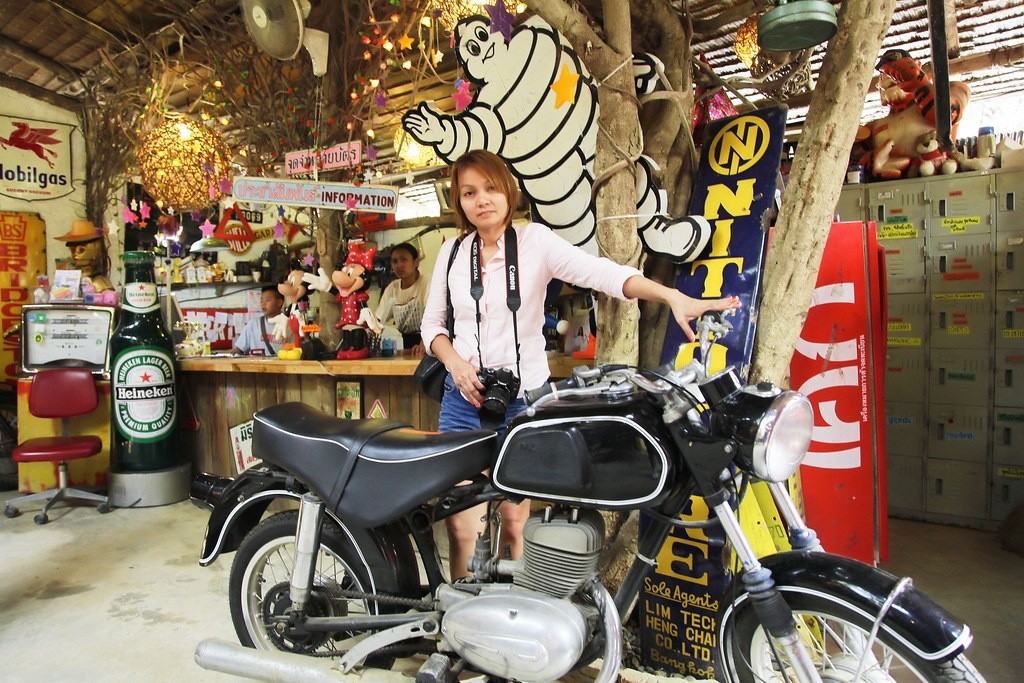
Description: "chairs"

(4, 367), (110, 525)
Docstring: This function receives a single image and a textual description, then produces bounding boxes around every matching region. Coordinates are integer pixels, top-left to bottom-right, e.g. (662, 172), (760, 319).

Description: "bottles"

(106, 249), (177, 472)
(845, 160), (865, 184)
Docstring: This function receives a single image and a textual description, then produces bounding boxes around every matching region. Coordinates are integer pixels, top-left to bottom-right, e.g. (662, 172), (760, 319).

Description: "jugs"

(977, 127), (996, 158)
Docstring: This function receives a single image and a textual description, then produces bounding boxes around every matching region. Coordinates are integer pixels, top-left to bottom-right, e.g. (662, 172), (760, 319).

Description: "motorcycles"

(194, 294), (994, 679)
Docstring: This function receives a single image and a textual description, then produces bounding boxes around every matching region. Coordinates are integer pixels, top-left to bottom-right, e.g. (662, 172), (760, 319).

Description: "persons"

(420, 149), (742, 582)
(373, 244), (430, 358)
(3, 221), (118, 339)
(231, 285), (294, 357)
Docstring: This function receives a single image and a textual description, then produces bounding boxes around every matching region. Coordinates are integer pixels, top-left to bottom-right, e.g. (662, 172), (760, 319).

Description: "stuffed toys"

(915, 130), (957, 176)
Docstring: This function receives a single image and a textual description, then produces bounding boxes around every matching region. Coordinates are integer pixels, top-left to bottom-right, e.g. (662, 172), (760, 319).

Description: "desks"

(176, 352), (440, 477)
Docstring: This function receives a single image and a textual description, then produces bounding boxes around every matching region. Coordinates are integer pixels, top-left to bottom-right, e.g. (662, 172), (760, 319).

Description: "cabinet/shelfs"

(831, 168), (1024, 534)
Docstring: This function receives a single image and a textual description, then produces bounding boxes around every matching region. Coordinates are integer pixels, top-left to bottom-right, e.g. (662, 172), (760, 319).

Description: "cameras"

(477, 367), (521, 431)
